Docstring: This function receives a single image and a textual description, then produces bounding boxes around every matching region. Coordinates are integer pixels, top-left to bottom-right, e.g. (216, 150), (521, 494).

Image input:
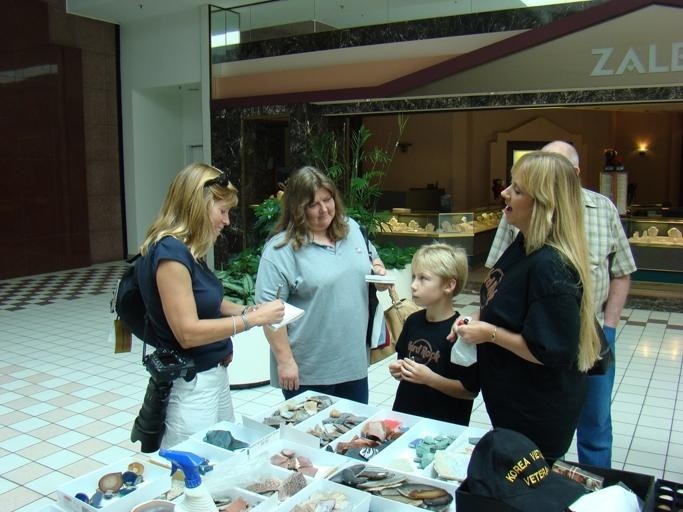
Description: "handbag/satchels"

(116, 264), (151, 345)
(359, 223), (391, 349)
(579, 312), (612, 374)
(369, 259), (423, 365)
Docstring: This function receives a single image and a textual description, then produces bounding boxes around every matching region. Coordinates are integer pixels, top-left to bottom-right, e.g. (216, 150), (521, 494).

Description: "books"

(268, 302), (305, 332)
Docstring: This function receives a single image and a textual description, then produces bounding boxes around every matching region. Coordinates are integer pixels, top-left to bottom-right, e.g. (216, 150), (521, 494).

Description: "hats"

(467, 428), (585, 511)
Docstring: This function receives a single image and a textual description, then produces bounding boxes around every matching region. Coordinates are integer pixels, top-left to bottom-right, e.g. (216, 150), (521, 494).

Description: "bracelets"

(242, 305), (252, 314)
(231, 315), (237, 337)
(240, 314), (250, 331)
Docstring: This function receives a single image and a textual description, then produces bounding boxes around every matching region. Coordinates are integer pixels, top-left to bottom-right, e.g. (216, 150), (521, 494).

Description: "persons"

(388, 244), (484, 426)
(255, 166), (392, 411)
(446, 150), (593, 469)
(139, 162), (284, 453)
(482, 138), (638, 471)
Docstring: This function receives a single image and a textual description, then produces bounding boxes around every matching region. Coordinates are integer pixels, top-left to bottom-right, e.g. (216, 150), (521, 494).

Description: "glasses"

(205, 172), (230, 189)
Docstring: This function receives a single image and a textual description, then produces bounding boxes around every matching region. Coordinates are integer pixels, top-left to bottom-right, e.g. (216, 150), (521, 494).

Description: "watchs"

(491, 328), (496, 343)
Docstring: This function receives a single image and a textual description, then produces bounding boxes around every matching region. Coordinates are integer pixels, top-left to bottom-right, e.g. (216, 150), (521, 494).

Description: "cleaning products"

(157, 449), (218, 511)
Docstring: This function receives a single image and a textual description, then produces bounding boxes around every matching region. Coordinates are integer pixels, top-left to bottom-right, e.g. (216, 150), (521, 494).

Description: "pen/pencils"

(276, 283), (284, 300)
(457, 318), (469, 326)
